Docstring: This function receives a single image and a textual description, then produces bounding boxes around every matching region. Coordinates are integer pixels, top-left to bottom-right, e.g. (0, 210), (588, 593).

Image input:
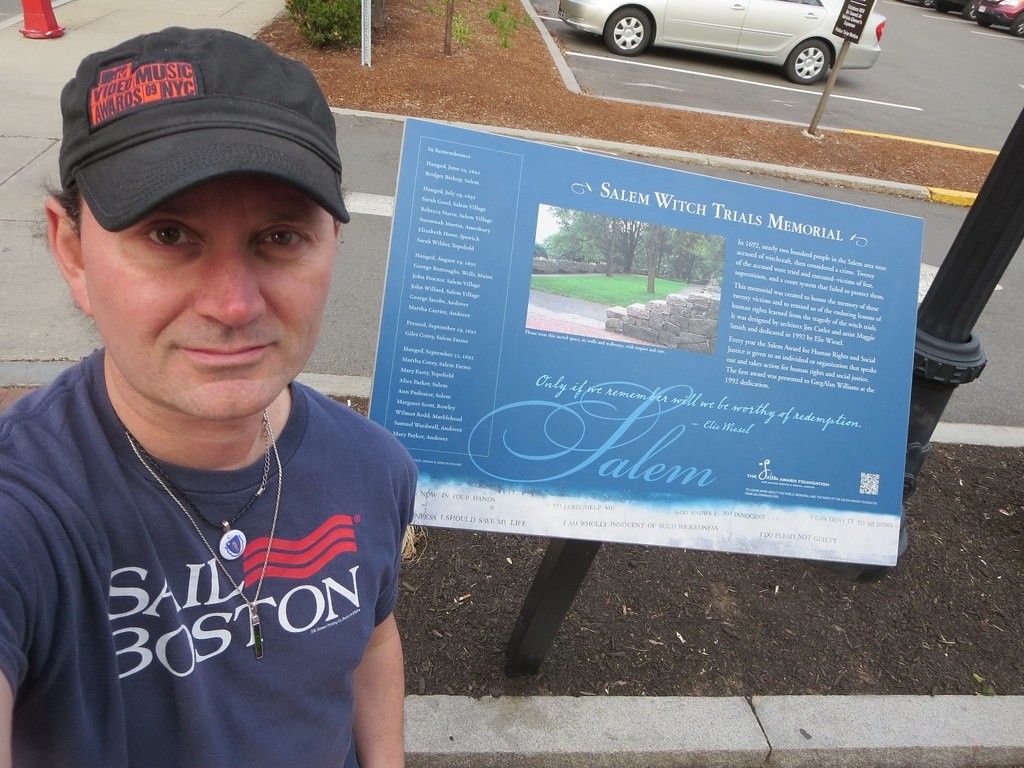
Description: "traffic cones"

(19, 0), (65, 39)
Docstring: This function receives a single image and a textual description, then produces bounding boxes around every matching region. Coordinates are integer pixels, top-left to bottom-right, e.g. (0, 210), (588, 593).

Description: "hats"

(59, 27), (350, 232)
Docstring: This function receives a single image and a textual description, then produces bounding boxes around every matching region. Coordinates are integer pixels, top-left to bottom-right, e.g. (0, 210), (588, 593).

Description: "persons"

(0, 29), (419, 766)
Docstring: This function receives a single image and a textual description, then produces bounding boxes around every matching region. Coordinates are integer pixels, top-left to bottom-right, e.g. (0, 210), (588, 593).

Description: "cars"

(558, 0), (886, 86)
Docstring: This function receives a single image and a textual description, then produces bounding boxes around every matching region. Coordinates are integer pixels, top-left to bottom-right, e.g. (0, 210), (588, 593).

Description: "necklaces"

(119, 423), (270, 560)
(123, 427), (282, 659)
